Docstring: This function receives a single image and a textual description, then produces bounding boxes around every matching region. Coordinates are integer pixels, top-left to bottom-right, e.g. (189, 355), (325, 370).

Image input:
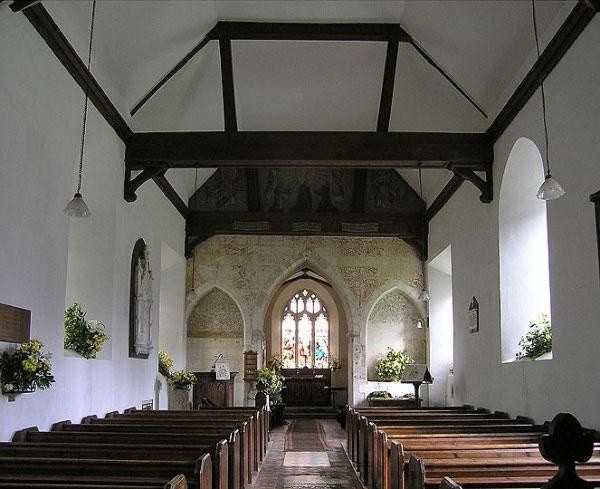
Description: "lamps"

(531, 0), (566, 201)
(187, 168), (199, 300)
(62, 0), (96, 218)
(418, 168), (430, 301)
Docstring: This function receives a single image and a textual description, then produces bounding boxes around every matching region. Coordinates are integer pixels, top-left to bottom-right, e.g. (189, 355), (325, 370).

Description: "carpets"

(283, 418), (333, 452)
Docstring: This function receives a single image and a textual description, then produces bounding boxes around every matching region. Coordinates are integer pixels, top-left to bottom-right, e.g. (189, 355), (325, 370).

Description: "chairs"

(345, 404), (600, 489)
(0, 404), (271, 489)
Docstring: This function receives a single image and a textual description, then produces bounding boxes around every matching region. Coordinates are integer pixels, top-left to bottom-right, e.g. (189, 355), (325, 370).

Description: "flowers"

(256, 353), (285, 395)
(158, 351), (198, 391)
(374, 349), (415, 381)
(0, 337), (56, 393)
(64, 302), (112, 361)
(515, 314), (552, 359)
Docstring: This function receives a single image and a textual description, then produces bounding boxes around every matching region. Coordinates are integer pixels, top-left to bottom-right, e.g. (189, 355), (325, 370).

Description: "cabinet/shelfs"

(367, 397), (423, 407)
